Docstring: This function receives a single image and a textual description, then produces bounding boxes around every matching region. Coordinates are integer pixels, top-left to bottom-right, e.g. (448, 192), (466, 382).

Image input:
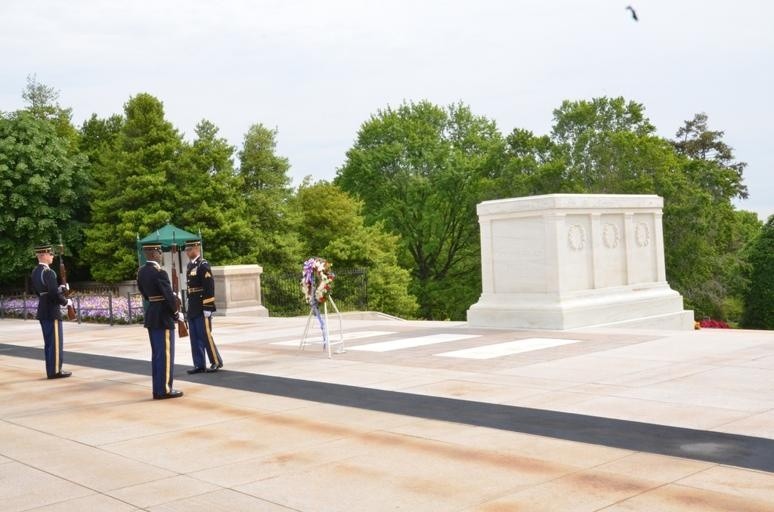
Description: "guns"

(55, 229), (77, 322)
(172, 231), (188, 338)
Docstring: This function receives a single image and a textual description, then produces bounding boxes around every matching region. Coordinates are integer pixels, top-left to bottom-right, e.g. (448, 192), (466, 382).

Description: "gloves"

(58, 283), (70, 292)
(178, 312), (184, 322)
(67, 299), (72, 306)
(203, 311), (211, 317)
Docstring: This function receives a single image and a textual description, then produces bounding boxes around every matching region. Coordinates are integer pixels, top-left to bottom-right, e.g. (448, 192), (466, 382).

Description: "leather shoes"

(153, 390), (182, 399)
(187, 363), (223, 374)
(48, 371), (72, 379)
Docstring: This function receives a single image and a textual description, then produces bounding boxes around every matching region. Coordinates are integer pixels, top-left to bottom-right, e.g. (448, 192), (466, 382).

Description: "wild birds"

(626, 6), (640, 22)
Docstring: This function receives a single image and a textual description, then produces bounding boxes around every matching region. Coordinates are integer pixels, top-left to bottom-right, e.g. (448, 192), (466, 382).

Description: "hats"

(185, 240), (200, 247)
(142, 244), (162, 249)
(34, 245), (54, 255)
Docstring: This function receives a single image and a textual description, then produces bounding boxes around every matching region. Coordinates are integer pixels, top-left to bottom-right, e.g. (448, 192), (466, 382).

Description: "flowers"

(301, 256), (337, 351)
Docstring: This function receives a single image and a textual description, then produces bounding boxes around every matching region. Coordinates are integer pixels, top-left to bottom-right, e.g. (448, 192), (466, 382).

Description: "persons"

(182, 239), (223, 373)
(135, 243), (184, 399)
(30, 244), (73, 380)
